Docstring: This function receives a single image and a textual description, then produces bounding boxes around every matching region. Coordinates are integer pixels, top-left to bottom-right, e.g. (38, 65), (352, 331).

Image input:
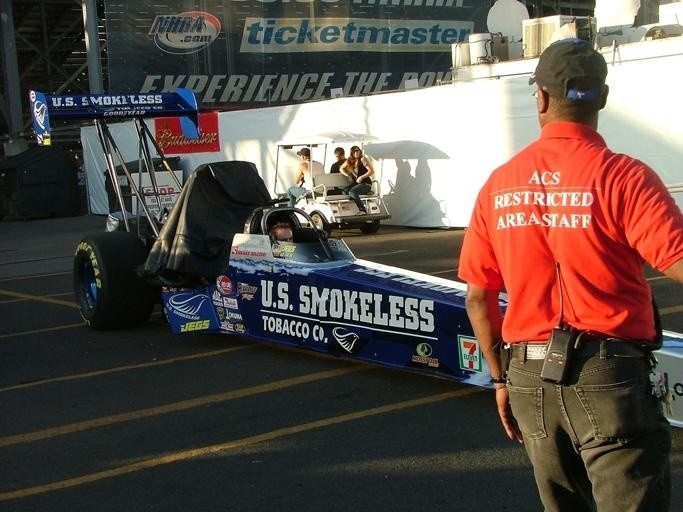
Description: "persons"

(285, 148), (324, 211)
(456, 37), (681, 511)
(330, 146), (347, 194)
(270, 222), (294, 244)
(339, 145), (374, 215)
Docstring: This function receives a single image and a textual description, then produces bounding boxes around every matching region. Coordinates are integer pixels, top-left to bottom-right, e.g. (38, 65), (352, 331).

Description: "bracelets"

(489, 374), (505, 384)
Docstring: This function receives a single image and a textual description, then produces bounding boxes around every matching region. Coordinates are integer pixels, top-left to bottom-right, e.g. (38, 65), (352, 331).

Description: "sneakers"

(355, 210), (367, 216)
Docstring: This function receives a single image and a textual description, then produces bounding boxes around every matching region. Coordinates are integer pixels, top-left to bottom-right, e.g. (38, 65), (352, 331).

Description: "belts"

(511, 343), (646, 360)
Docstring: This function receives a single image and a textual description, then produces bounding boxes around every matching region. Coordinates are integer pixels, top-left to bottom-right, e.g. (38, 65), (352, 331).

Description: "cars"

(639, 23), (683, 42)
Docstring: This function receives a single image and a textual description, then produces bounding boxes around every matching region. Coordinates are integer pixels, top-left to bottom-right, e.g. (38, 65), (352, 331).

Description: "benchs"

(312, 172), (379, 218)
(293, 194), (349, 218)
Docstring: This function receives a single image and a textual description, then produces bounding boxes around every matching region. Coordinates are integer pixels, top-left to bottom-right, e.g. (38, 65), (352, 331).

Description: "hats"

(528, 37), (608, 101)
(296, 147), (310, 157)
